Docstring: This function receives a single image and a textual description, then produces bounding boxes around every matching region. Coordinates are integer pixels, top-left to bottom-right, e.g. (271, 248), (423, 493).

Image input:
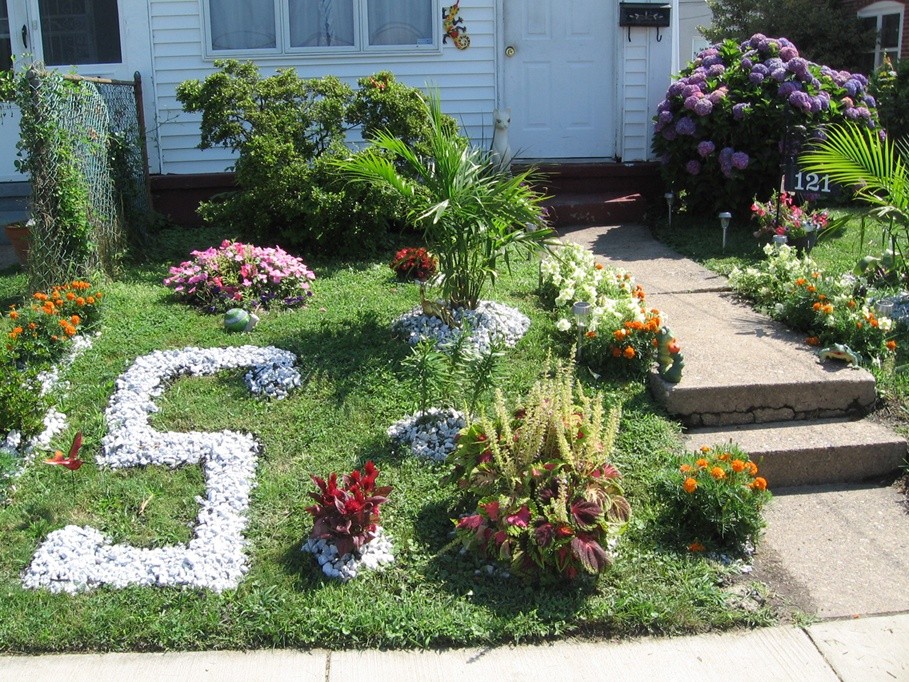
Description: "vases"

(787, 230), (817, 259)
(6, 220), (36, 264)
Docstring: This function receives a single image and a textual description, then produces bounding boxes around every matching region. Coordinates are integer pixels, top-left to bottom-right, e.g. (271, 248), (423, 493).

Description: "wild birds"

(419, 286), (451, 325)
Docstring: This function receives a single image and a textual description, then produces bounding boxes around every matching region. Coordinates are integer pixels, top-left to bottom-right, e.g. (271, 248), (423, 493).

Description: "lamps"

(572, 301), (590, 328)
(773, 235), (788, 257)
(874, 299), (894, 320)
(718, 212), (731, 229)
(525, 222), (537, 235)
(665, 192), (675, 206)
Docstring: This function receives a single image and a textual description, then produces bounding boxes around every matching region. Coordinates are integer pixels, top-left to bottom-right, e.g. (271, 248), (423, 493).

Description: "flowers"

(751, 190), (828, 240)
